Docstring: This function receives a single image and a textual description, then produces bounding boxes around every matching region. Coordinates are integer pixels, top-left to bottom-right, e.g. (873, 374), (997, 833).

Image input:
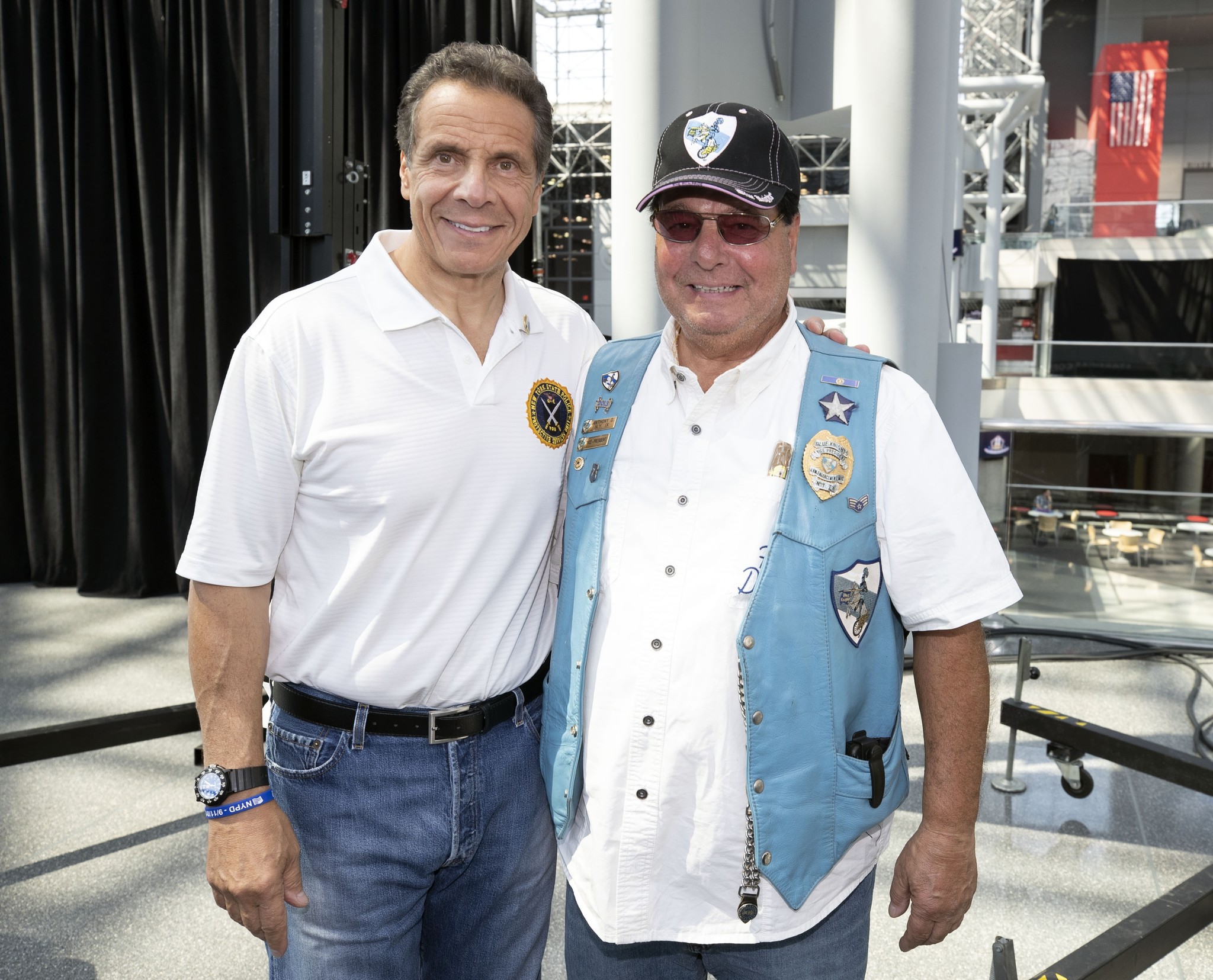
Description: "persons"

(175, 42), (872, 979)
(532, 100), (1023, 980)
(1032, 489), (1052, 545)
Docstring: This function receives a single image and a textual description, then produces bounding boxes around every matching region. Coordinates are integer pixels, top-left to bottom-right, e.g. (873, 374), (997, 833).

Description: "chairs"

(1011, 506), (1213, 586)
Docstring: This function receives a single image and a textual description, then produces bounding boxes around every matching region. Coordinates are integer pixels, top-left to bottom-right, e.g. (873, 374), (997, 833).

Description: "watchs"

(194, 764), (270, 809)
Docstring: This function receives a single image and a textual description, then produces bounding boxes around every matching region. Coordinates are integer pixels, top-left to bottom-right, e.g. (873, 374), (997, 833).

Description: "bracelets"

(206, 791), (274, 818)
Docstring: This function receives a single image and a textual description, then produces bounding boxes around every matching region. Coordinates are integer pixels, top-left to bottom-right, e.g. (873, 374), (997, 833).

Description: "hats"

(635, 102), (801, 212)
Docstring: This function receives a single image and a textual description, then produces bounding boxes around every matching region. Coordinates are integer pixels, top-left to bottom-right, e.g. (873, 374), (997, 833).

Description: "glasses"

(650, 201), (788, 247)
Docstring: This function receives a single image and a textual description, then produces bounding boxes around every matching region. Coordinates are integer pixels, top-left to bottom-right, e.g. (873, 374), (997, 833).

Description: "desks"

(1102, 527), (1144, 564)
(1027, 509), (1062, 546)
(1177, 522), (1213, 545)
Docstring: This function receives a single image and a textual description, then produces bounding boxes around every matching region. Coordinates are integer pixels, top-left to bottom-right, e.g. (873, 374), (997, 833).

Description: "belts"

(272, 651), (553, 744)
